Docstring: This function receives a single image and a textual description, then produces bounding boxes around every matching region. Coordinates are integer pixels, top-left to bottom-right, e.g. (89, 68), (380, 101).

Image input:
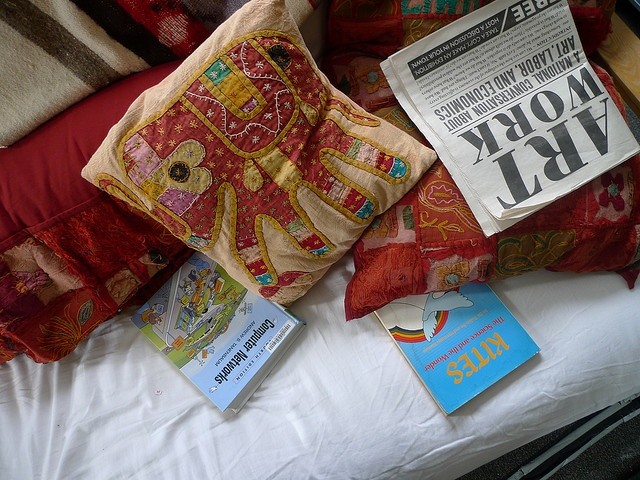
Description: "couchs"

(0, 1), (640, 479)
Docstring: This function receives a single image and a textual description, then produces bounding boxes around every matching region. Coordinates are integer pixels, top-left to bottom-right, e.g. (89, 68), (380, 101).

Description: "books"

(128, 250), (307, 414)
(373, 281), (542, 418)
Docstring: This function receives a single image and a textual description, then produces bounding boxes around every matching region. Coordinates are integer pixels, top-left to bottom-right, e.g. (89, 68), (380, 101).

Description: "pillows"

(322, 1), (640, 322)
(80, 0), (438, 307)
(0, 58), (185, 367)
